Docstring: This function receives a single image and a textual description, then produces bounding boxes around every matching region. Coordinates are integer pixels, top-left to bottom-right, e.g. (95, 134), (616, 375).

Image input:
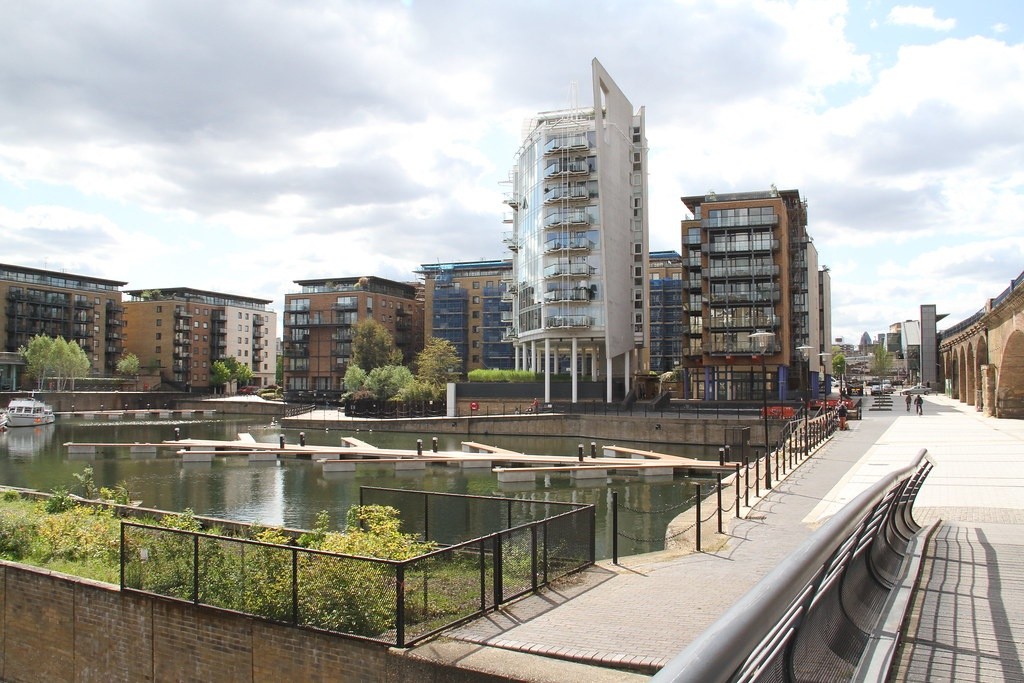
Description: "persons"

(906, 394), (911, 410)
(531, 398), (538, 413)
(914, 394), (923, 415)
(837, 405), (848, 431)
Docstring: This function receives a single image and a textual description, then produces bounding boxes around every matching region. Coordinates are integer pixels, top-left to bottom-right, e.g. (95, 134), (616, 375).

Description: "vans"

(237, 384), (259, 396)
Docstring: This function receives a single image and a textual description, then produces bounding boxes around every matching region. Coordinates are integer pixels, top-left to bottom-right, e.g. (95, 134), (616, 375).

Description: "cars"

(831, 376), (905, 397)
(902, 385), (933, 395)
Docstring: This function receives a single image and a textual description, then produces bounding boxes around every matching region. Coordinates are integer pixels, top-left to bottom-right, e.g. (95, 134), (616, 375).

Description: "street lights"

(795, 338), (815, 456)
(817, 350), (832, 439)
(747, 327), (776, 489)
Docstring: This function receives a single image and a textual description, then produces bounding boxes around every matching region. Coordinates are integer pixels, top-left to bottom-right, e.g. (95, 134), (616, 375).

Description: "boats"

(7, 397), (56, 427)
(0, 405), (12, 432)
